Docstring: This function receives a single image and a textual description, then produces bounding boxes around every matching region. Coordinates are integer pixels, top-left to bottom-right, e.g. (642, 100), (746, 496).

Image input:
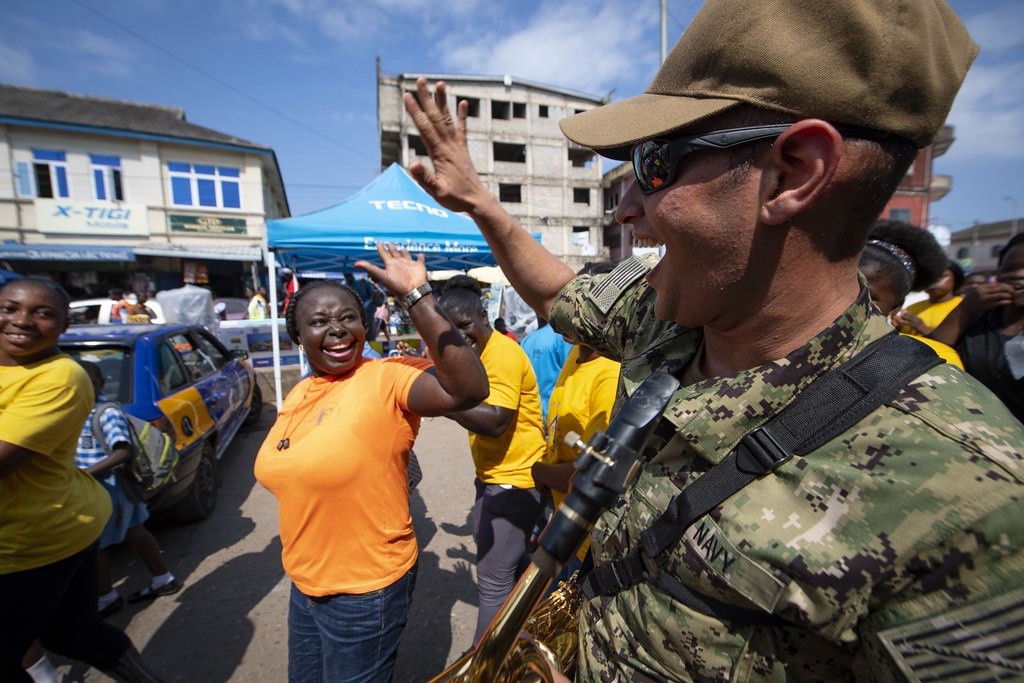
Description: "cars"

(56, 324), (264, 525)
(67, 298), (165, 324)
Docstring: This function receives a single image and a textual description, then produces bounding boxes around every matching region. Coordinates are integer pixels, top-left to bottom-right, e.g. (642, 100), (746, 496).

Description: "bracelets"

(399, 282), (433, 309)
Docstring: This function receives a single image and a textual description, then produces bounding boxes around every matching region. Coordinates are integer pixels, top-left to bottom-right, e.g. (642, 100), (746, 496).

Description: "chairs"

(97, 356), (122, 399)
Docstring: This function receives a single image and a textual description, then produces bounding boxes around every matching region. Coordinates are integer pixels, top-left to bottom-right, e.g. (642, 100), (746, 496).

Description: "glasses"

(631, 123), (794, 194)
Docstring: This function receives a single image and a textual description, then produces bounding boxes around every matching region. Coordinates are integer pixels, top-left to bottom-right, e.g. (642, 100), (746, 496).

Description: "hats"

(558, 0), (981, 150)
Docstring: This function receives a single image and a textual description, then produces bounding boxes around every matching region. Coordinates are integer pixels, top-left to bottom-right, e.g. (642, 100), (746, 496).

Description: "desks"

(377, 335), (426, 355)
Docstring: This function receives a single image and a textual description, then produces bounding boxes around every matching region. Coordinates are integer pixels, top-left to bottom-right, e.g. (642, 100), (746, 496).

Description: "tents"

(266, 162), (542, 414)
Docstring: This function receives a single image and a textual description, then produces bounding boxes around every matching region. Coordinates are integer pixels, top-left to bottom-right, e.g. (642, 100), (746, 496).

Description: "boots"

(105, 645), (166, 683)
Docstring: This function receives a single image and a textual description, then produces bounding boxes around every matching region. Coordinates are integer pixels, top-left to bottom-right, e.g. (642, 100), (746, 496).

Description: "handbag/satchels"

(92, 403), (154, 496)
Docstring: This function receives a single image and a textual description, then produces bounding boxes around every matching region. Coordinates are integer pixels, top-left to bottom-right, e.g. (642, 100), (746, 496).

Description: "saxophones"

(426, 371), (680, 683)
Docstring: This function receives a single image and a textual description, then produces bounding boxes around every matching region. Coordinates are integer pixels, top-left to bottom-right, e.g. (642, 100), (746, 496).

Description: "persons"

(859, 218), (1024, 425)
(97, 285), (166, 325)
(362, 301), (433, 360)
(253, 241), (490, 683)
(75, 360), (178, 620)
(0, 278), (168, 683)
(437, 261), (629, 656)
(242, 268), (300, 320)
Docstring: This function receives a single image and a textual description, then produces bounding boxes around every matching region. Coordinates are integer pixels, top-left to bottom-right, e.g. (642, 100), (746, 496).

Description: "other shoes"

(127, 578), (178, 604)
(97, 595), (123, 618)
